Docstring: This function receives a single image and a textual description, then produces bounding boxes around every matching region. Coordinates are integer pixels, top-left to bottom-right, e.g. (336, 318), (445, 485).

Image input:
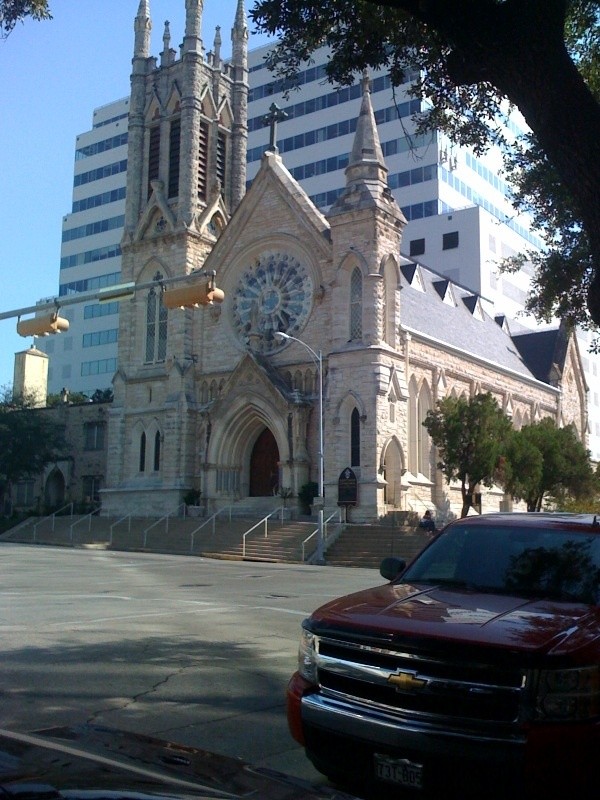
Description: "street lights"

(273, 331), (324, 497)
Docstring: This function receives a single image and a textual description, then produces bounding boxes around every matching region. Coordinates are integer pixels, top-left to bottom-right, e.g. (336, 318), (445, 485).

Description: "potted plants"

(299, 480), (318, 515)
(183, 487), (205, 519)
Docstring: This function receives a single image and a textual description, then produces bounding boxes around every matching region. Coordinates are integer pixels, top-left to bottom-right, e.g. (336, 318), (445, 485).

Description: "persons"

(270, 466), (278, 496)
(419, 510), (434, 528)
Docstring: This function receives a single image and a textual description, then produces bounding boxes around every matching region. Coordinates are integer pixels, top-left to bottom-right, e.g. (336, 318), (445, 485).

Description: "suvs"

(285, 510), (599, 800)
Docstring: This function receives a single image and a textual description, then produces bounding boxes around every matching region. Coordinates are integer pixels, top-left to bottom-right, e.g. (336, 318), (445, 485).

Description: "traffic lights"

(164, 279), (225, 312)
(17, 312), (70, 339)
